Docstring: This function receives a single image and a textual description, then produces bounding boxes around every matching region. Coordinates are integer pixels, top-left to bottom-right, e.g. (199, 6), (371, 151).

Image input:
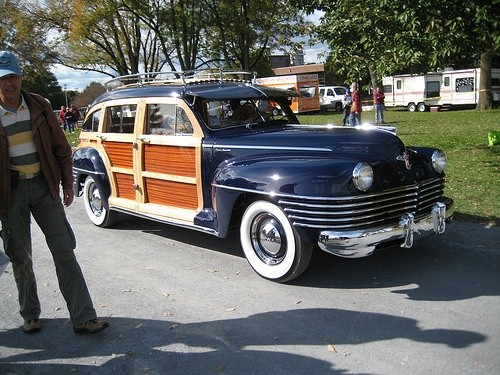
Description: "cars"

(67, 71), (456, 283)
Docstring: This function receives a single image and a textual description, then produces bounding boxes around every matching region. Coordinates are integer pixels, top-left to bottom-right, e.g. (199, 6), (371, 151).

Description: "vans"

(320, 87), (351, 113)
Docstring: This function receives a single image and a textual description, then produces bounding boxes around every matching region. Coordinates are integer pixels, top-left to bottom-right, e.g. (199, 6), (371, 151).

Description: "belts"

(8, 171), (43, 179)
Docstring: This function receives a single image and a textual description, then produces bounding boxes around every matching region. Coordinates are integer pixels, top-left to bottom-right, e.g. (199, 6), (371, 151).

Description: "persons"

(373, 87), (385, 124)
(342, 82), (362, 126)
(0, 50), (110, 334)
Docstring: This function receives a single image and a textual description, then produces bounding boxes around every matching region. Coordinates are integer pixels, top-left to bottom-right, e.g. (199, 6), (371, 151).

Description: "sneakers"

(22, 319), (42, 333)
(73, 319), (109, 334)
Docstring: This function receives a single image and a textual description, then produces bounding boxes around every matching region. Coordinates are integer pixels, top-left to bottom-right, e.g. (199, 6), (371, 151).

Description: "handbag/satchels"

(488, 131), (500, 145)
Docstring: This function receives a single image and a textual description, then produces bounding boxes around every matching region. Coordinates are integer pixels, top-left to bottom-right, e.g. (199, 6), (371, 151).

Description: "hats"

(0, 50), (21, 77)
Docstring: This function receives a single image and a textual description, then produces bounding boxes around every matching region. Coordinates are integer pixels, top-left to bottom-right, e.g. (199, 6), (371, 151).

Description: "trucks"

(380, 73), (439, 112)
(440, 69), (500, 112)
(250, 74), (320, 114)
